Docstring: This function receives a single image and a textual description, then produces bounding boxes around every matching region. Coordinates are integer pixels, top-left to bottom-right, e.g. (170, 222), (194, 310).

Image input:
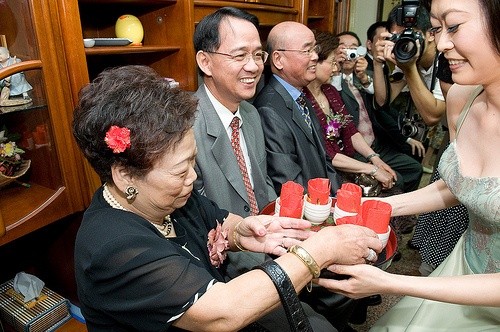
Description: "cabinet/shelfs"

(0, 0), (350, 332)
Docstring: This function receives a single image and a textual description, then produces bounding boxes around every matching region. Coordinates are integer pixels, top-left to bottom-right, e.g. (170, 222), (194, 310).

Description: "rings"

(366, 248), (377, 263)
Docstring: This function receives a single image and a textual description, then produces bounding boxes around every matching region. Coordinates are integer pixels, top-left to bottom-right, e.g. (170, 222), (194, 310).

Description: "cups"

(274, 196), (306, 219)
(378, 225), (391, 251)
(304, 193), (332, 225)
(333, 201), (358, 225)
(83, 38), (95, 47)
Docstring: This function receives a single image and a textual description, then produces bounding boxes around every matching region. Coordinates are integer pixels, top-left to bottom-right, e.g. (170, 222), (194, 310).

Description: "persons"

(302, 0), (500, 332)
(0, 47), (33, 99)
(251, 0), (457, 332)
(72, 65), (383, 332)
(182, 7), (279, 220)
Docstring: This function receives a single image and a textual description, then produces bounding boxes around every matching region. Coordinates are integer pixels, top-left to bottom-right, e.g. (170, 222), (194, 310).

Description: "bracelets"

(232, 222), (248, 254)
(369, 166), (379, 177)
(287, 244), (321, 281)
(364, 152), (380, 161)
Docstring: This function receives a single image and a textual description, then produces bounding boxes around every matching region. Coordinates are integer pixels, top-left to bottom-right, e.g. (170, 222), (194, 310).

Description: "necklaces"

(103, 184), (174, 237)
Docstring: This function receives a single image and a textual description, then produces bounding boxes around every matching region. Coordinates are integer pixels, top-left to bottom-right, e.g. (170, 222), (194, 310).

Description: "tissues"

(0, 270), (72, 332)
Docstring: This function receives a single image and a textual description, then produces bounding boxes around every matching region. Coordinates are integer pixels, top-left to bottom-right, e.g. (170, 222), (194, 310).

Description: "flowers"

(206, 218), (231, 269)
(0, 128), (27, 176)
(323, 107), (354, 141)
(105, 123), (133, 154)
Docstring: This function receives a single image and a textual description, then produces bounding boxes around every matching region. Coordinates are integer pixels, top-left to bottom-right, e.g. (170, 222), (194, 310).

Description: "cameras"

(403, 117), (428, 143)
(342, 49), (359, 59)
(384, 0), (423, 62)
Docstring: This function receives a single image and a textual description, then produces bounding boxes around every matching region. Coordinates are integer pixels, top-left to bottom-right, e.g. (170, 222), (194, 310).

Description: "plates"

(92, 38), (133, 45)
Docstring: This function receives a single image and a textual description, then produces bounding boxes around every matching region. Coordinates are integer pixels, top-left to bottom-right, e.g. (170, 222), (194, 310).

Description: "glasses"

(207, 50), (269, 66)
(322, 58), (337, 68)
(269, 44), (320, 58)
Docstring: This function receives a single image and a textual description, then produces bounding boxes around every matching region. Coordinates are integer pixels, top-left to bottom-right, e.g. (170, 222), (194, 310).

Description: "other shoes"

(367, 294), (382, 306)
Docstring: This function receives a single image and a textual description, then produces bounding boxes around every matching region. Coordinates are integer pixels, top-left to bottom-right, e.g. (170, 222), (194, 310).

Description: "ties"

(295, 95), (313, 136)
(227, 117), (259, 216)
